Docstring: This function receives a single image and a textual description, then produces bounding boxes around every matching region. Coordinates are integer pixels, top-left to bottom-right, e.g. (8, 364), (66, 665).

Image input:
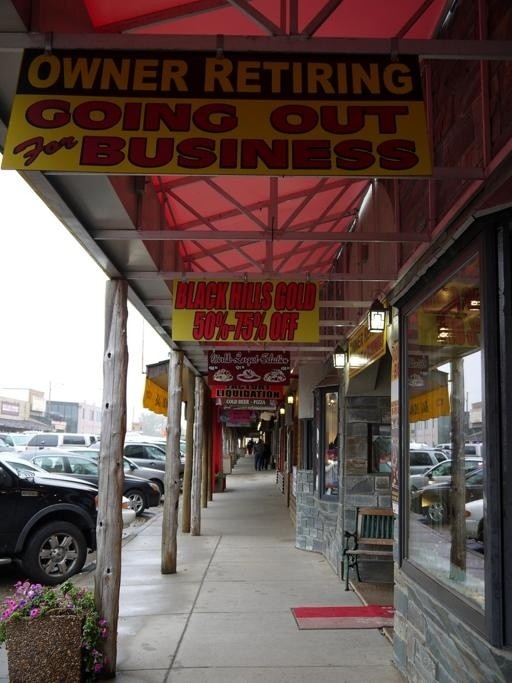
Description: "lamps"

(366, 297), (392, 333)
(287, 389), (297, 404)
(279, 404), (285, 416)
(332, 345), (349, 368)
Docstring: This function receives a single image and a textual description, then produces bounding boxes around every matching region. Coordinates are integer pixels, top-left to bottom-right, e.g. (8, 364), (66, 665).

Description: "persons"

(247, 438), (272, 471)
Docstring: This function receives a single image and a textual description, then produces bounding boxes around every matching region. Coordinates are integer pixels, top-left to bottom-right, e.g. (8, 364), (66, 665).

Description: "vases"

(5, 616), (85, 683)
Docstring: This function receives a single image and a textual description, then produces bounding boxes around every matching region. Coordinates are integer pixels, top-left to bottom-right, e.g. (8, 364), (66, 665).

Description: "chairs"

(72, 463), (94, 474)
(42, 459), (63, 473)
(339, 505), (395, 591)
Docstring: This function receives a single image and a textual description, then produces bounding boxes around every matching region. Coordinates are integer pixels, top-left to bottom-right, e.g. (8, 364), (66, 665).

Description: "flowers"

(0, 577), (112, 676)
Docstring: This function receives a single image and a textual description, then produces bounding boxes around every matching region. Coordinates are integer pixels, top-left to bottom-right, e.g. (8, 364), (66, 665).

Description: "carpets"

(290, 605), (395, 631)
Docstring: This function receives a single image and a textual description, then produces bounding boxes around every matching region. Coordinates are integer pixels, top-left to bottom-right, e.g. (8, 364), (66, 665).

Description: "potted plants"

(215, 471), (226, 492)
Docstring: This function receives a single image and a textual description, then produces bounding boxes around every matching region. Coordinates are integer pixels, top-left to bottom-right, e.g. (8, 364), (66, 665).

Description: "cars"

(409, 443), (485, 544)
(0, 431), (187, 587)
